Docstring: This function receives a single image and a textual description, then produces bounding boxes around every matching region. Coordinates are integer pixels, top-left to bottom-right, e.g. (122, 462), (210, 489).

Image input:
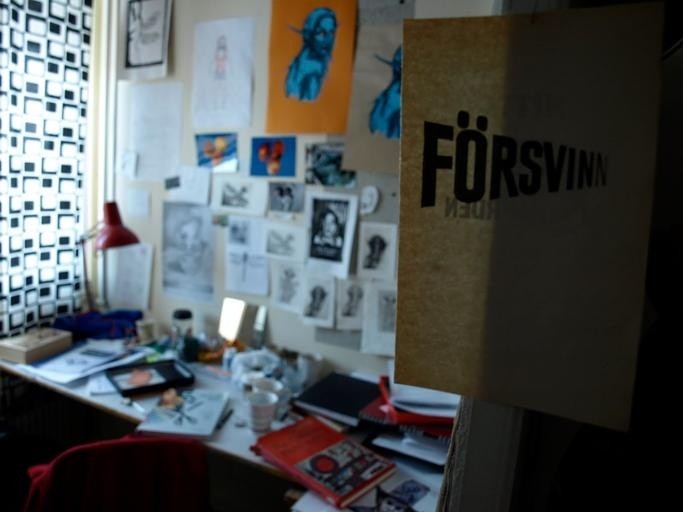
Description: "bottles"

(169, 309), (193, 349)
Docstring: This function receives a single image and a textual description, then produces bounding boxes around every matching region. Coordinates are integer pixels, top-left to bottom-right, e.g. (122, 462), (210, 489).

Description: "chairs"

(24, 434), (210, 512)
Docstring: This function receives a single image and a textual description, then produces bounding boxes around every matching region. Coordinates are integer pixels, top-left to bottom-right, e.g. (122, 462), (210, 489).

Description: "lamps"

(74, 201), (140, 319)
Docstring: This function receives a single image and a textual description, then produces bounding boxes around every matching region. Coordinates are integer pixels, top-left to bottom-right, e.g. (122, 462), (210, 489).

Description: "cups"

(244, 378), (283, 440)
(297, 352), (323, 390)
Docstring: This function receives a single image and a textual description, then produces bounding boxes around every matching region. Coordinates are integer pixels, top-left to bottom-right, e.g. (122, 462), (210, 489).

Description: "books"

(136, 385), (230, 440)
(282, 371), (463, 445)
(248, 416), (399, 509)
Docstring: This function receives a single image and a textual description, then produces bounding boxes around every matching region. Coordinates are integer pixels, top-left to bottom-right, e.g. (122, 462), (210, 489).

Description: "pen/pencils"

(217, 409), (233, 429)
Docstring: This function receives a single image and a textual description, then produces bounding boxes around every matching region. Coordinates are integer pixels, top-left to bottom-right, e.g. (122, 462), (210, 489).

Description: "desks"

(1, 336), (440, 511)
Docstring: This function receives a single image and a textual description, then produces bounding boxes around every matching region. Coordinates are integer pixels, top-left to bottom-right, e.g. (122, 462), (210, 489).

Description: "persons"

(313, 208), (342, 248)
